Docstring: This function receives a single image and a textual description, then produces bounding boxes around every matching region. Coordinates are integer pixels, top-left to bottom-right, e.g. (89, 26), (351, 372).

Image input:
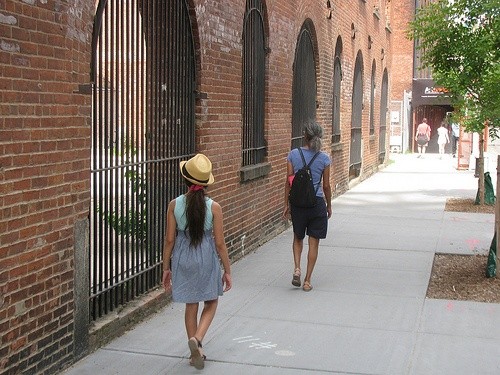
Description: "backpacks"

(289, 147), (324, 207)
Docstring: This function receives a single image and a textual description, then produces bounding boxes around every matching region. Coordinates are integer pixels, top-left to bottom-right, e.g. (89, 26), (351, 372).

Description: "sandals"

(188, 337), (206, 369)
(292, 270), (301, 287)
(303, 282), (313, 291)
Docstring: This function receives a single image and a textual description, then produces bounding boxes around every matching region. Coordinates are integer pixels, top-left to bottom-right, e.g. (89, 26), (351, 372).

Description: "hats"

(180, 154), (215, 186)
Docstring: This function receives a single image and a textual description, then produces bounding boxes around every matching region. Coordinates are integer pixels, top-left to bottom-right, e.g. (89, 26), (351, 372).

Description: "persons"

(282, 124), (332, 292)
(415, 118), (431, 159)
(451, 123), (460, 158)
(162, 154), (232, 371)
(437, 121), (450, 160)
